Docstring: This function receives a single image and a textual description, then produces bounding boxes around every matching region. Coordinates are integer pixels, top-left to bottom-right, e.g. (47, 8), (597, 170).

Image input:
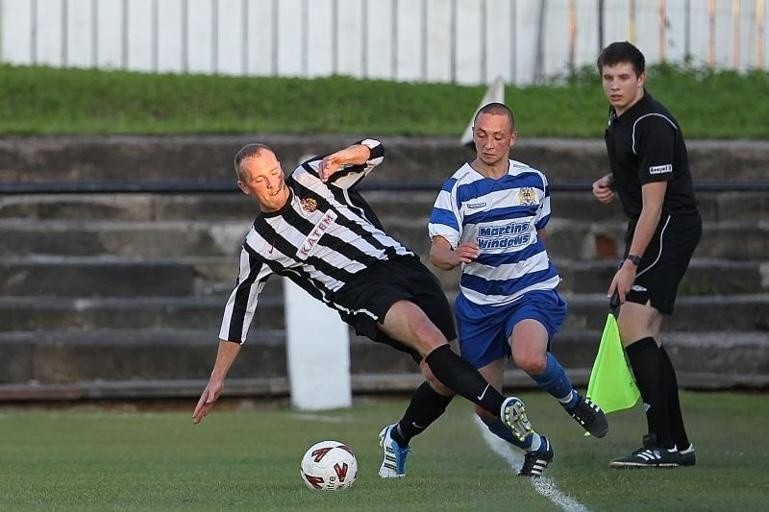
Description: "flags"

(582, 312), (640, 437)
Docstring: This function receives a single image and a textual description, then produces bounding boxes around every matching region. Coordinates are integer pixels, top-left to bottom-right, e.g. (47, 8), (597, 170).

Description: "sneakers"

(557, 387), (609, 439)
(378, 422), (411, 478)
(500, 395), (532, 443)
(677, 440), (696, 466)
(607, 433), (680, 469)
(517, 434), (553, 478)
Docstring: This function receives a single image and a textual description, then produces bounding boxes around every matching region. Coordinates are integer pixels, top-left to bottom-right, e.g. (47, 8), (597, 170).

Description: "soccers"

(300, 440), (358, 492)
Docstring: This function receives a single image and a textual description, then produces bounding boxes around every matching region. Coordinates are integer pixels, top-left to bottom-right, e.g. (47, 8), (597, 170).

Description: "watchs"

(627, 254), (641, 265)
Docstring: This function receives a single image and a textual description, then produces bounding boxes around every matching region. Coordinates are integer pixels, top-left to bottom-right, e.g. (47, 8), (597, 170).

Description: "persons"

(590, 40), (704, 470)
(190, 135), (533, 478)
(425, 101), (612, 480)
